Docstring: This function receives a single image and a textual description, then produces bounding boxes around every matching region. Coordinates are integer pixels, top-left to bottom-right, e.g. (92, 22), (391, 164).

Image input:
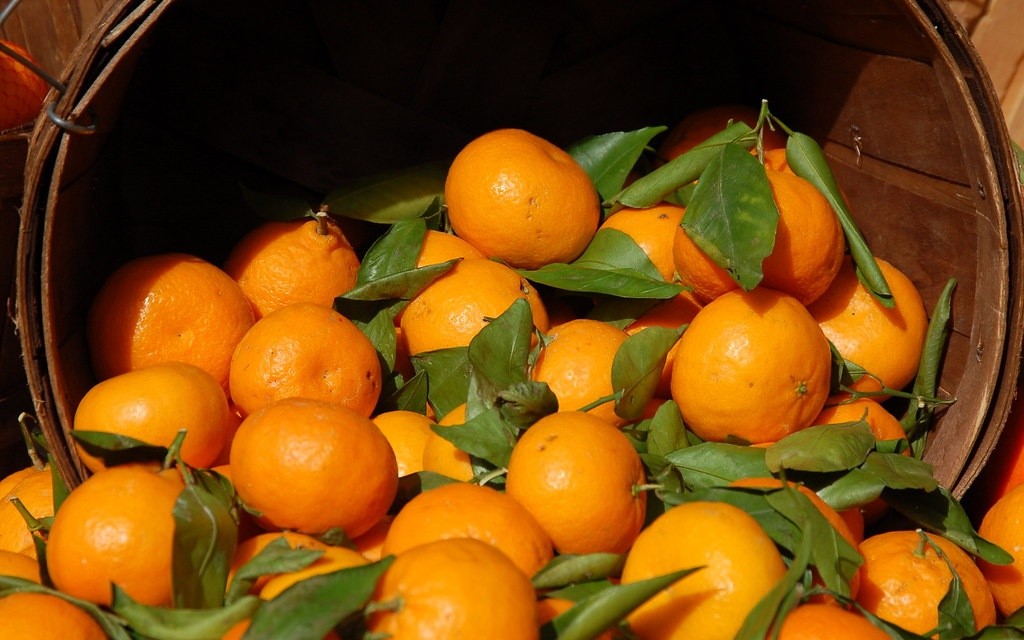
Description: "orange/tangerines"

(0, 102), (1024, 639)
